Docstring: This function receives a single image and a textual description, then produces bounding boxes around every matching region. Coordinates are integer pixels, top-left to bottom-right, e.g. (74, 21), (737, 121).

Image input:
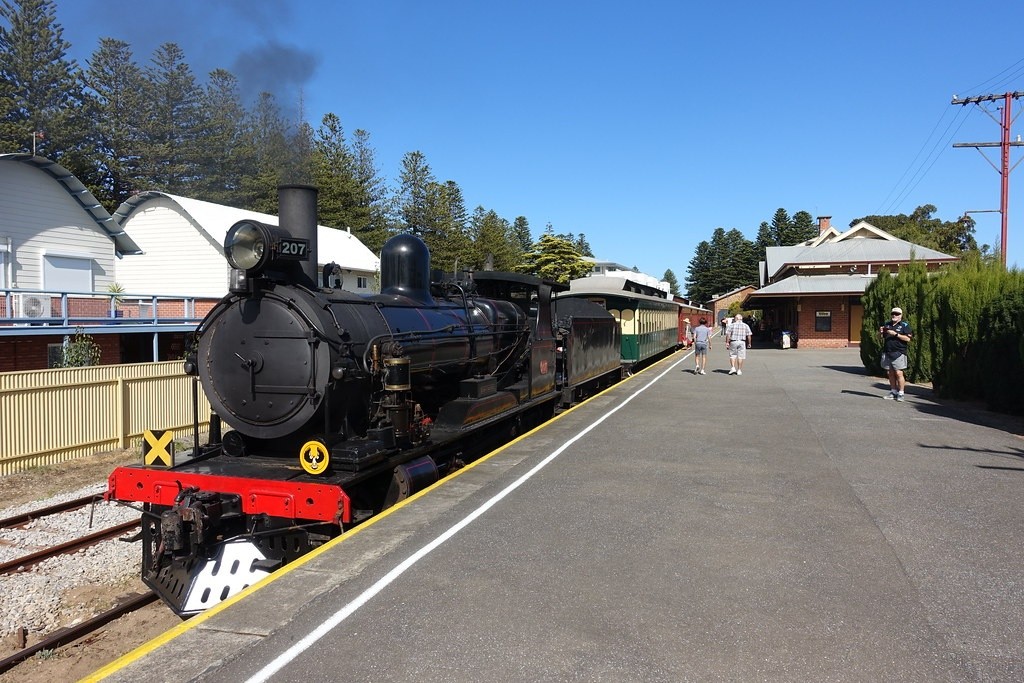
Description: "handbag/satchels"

(690, 328), (694, 334)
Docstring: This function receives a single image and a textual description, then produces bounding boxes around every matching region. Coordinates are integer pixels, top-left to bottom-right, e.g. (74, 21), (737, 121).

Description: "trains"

(102, 184), (714, 616)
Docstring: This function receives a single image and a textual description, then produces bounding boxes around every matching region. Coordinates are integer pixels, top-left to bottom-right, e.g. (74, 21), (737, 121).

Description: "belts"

(730, 340), (745, 341)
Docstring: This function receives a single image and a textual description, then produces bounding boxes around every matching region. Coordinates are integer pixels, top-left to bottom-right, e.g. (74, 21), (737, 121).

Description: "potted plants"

(106, 282), (125, 325)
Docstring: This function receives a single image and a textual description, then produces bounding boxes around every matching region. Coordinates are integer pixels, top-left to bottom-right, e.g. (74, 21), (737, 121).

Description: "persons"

(682, 318), (692, 350)
(693, 317), (712, 375)
(880, 308), (912, 402)
(725, 314), (752, 376)
(721, 315), (773, 338)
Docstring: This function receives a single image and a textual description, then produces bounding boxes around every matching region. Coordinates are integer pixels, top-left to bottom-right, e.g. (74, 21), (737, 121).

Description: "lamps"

(841, 304), (844, 312)
(797, 305), (801, 311)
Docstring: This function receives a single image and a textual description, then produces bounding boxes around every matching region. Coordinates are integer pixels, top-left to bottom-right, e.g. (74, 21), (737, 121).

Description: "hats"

(683, 318), (691, 323)
(892, 307), (902, 313)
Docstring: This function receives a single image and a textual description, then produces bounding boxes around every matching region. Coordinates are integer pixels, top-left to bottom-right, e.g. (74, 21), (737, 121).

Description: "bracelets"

(894, 333), (898, 336)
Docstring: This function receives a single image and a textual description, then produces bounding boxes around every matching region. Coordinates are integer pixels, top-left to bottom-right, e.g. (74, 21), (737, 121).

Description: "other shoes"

(897, 394), (904, 402)
(681, 347), (687, 350)
(688, 347), (691, 350)
(700, 370), (706, 375)
(729, 368), (736, 374)
(883, 392), (898, 399)
(694, 365), (700, 375)
(737, 370), (742, 375)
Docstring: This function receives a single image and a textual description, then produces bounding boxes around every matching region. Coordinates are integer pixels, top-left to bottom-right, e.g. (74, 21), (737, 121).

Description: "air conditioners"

(12, 293), (51, 319)
